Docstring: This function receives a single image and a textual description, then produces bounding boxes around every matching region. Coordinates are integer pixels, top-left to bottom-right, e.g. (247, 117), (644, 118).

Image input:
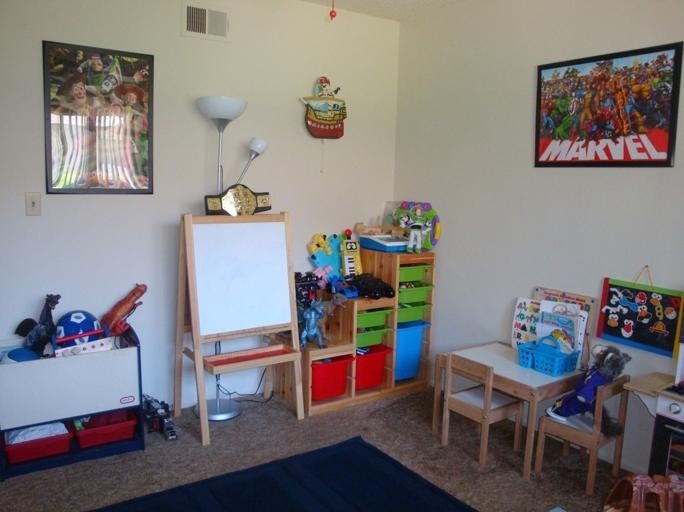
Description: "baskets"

(516, 336), (580, 378)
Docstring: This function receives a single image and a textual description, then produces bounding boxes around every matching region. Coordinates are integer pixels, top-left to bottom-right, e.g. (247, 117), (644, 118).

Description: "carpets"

(94, 436), (478, 510)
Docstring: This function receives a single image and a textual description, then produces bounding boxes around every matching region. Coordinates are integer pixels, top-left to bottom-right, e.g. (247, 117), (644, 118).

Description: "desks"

(431, 342), (588, 482)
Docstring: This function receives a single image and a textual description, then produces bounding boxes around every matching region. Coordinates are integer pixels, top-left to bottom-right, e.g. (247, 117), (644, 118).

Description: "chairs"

(534, 374), (631, 498)
(442, 349), (526, 465)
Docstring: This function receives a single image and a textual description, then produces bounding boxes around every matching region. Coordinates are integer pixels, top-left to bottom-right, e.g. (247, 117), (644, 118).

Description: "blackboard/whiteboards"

(184, 212), (299, 345)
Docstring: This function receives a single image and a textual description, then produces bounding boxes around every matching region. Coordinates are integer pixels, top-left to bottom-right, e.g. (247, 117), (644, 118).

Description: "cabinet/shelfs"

(647, 383), (684, 479)
(277, 236), (436, 418)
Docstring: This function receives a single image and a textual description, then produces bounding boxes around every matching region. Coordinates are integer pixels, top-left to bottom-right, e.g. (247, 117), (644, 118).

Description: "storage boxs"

(311, 354), (355, 403)
(395, 320), (432, 384)
(0, 325), (145, 477)
(396, 302), (433, 322)
(399, 264), (433, 287)
(397, 285), (433, 306)
(4, 421), (75, 465)
(355, 327), (395, 348)
(352, 344), (393, 395)
(74, 409), (140, 450)
(356, 308), (393, 329)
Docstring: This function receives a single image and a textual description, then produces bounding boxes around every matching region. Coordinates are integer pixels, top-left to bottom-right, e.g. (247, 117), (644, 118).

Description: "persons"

(49, 45), (149, 189)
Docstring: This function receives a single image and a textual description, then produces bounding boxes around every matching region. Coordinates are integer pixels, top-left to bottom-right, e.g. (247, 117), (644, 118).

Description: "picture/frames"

(41, 39), (158, 198)
(534, 41), (684, 168)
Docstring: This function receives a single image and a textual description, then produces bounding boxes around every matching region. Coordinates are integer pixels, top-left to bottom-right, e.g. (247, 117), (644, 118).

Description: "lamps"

(194, 93), (269, 422)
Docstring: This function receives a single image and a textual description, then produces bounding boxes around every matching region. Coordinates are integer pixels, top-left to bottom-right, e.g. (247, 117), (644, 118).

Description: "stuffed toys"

(14, 293), (62, 355)
